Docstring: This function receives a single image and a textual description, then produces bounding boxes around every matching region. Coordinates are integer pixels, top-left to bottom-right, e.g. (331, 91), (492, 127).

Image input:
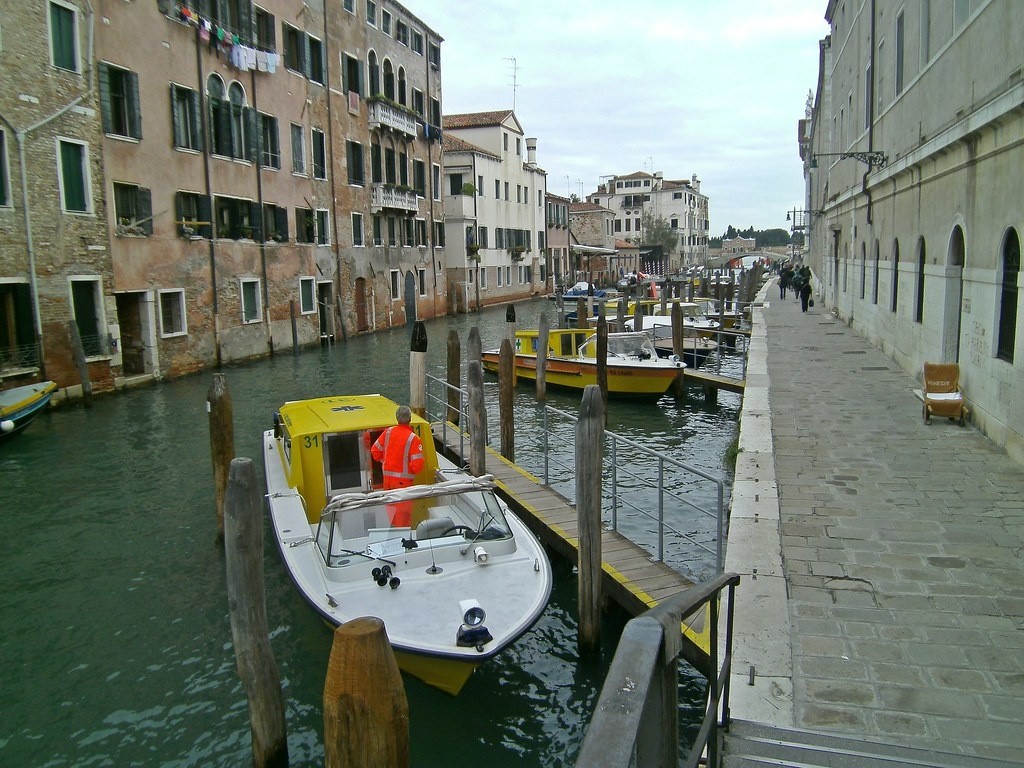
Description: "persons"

(782, 263), (812, 303)
(633, 266), (637, 278)
(619, 266), (624, 279)
(370, 406), (426, 527)
(798, 278), (812, 313)
(778, 270), (787, 301)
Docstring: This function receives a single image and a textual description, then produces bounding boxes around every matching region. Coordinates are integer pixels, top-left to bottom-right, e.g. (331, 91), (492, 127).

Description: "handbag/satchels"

(809, 299), (814, 307)
(777, 278), (781, 285)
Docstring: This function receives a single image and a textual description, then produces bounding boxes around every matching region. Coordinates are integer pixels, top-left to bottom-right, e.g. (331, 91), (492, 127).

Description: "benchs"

(428, 504), (478, 534)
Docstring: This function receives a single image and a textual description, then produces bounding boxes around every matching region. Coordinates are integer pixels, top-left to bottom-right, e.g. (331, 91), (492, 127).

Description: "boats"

(0, 380), (62, 440)
(561, 266), (745, 370)
(481, 327), (687, 400)
(263, 393), (554, 698)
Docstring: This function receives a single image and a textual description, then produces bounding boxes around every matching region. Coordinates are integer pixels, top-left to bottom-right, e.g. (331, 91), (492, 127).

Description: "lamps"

(786, 210), (825, 222)
(809, 151), (888, 172)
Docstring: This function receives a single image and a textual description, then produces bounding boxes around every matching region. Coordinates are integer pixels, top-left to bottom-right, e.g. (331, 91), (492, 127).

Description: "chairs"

(922, 362), (969, 426)
